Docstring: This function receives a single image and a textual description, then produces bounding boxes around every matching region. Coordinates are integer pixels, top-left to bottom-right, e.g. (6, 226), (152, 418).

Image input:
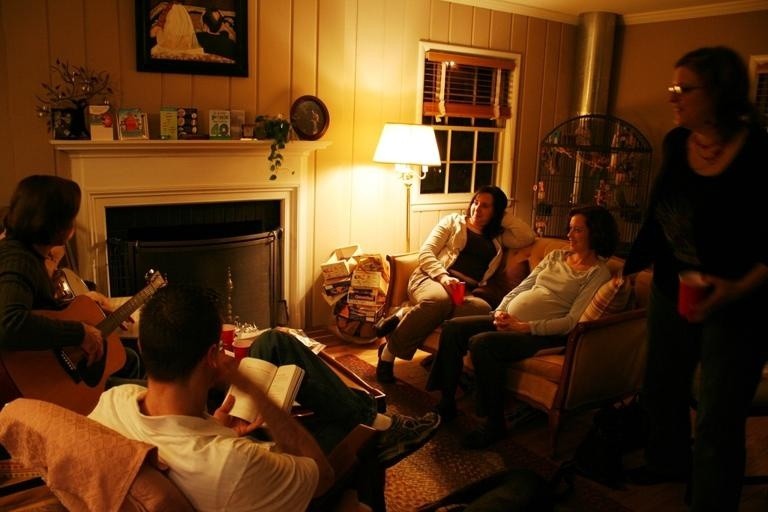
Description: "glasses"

(665, 83), (702, 100)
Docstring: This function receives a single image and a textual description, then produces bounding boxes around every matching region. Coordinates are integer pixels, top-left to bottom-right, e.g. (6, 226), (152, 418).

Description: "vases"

(73, 112), (92, 140)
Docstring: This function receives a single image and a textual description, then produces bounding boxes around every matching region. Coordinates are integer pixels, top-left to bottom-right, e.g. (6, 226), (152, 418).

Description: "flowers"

(33, 57), (117, 134)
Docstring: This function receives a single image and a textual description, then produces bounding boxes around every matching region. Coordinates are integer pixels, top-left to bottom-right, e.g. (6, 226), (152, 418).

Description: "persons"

(623, 46), (768, 512)
(192, 9), (236, 58)
(86, 284), (443, 512)
(0, 175), (148, 392)
(426, 206), (619, 451)
(373, 186), (535, 385)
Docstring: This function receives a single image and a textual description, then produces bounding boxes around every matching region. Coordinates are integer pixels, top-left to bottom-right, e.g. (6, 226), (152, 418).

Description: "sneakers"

(371, 410), (442, 469)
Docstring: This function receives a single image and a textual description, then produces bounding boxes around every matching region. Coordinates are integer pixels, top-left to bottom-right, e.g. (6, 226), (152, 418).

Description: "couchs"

(383, 238), (657, 460)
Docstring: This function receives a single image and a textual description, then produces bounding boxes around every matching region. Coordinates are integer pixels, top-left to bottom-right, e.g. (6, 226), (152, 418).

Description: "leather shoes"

(375, 316), (400, 337)
(433, 403), (457, 419)
(462, 425), (492, 449)
(375, 342), (396, 384)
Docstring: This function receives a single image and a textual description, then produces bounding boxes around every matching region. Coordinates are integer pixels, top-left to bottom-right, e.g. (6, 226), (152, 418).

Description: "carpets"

(332, 352), (628, 512)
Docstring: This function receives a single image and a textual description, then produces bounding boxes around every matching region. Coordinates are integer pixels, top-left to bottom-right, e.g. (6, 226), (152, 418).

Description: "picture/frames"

(118, 112), (151, 144)
(134, 0), (249, 77)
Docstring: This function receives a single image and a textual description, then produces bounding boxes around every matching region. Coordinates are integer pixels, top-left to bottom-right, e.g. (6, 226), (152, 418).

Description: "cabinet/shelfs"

(45, 140), (332, 332)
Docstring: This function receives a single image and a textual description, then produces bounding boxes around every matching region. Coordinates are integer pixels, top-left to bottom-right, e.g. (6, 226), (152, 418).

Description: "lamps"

(372, 124), (441, 255)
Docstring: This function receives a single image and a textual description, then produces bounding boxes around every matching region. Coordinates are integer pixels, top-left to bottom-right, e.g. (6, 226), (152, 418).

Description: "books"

(222, 357), (306, 429)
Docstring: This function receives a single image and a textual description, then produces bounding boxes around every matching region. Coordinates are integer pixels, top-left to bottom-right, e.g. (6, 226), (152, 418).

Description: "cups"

(679, 273), (706, 320)
(222, 324), (235, 348)
(450, 281), (466, 305)
(232, 340), (252, 363)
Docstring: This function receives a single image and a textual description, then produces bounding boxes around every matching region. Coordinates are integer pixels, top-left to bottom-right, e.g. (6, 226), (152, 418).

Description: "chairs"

(0, 398), (196, 512)
